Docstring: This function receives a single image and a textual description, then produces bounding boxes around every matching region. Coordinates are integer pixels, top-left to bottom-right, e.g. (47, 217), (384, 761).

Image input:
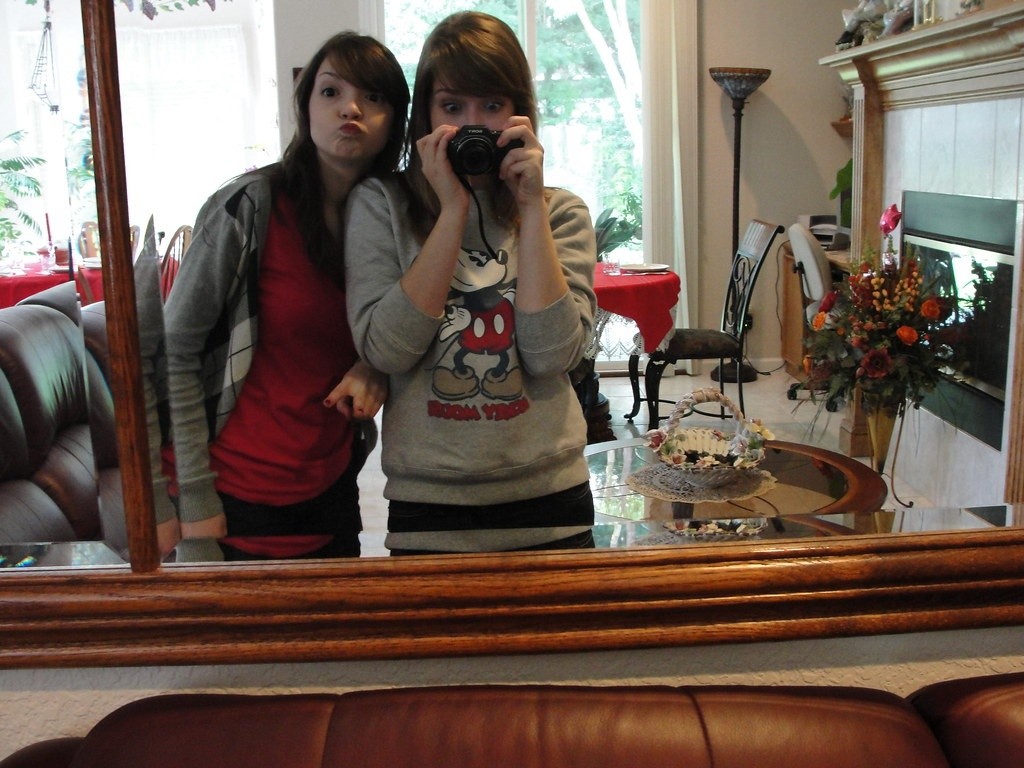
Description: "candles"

(45, 214), (51, 242)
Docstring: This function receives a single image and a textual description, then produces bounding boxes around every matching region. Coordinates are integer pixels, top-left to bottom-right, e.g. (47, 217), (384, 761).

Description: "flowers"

(789, 199), (982, 448)
(644, 426), (766, 471)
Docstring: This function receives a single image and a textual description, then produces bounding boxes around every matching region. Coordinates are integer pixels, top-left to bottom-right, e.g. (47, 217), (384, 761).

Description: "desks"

(778, 240), (854, 390)
(589, 260), (681, 419)
(1, 256), (180, 305)
(581, 435), (889, 516)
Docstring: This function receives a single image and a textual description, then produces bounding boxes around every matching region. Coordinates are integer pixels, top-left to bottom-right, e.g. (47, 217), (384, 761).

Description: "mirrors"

(0, 0), (1023, 662)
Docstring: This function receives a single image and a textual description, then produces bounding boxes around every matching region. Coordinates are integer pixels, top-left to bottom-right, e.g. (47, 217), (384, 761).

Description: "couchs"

(0, 671), (1023, 768)
(1, 278), (131, 569)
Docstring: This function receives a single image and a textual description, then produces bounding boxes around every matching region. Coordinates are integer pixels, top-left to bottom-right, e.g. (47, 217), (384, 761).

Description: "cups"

(602, 253), (621, 276)
(55, 248), (69, 265)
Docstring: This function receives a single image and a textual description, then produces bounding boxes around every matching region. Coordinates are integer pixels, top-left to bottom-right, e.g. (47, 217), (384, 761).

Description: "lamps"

(708, 67), (772, 382)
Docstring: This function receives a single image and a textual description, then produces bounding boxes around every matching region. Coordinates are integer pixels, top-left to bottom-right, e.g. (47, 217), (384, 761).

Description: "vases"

(857, 379), (901, 475)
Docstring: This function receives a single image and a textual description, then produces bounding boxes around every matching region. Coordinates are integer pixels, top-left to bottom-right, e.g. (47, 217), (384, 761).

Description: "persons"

(171, 538), (360, 562)
(162, 32), (411, 537)
(383, 532), (595, 556)
(342, 10), (596, 532)
(133, 222), (181, 553)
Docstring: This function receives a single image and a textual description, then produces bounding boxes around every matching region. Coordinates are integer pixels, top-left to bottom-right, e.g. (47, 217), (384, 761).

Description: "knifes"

(622, 272), (669, 276)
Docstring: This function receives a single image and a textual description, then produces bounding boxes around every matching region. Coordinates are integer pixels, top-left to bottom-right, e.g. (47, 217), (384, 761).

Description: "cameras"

(447, 124), (524, 176)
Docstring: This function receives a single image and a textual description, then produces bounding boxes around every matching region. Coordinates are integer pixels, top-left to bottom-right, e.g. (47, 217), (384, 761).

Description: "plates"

(620, 264), (669, 272)
(83, 256), (102, 269)
(49, 265), (69, 273)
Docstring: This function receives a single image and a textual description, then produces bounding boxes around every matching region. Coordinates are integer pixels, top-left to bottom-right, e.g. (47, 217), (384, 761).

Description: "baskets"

(642, 386), (777, 487)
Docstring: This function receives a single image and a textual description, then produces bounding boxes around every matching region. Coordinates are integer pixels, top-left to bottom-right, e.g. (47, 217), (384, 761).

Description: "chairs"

(160, 223), (192, 304)
(787, 220), (854, 332)
(624, 220), (781, 432)
(76, 222), (139, 261)
